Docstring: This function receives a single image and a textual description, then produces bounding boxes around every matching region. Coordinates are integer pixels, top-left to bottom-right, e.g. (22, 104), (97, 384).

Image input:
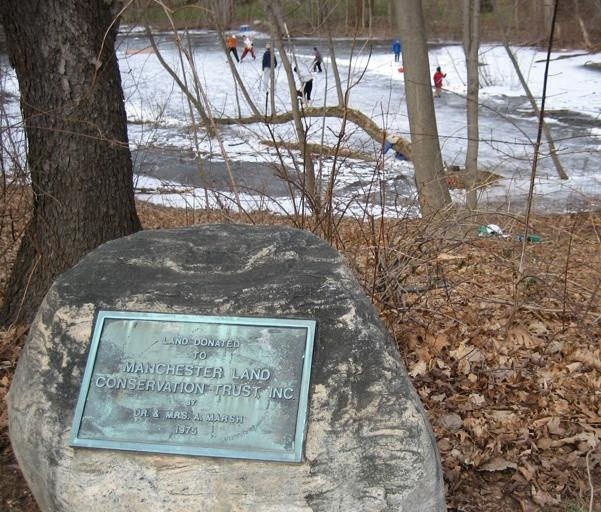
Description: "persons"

(226, 33), (256, 63)
(392, 40), (401, 62)
(434, 67), (446, 98)
(263, 44), (277, 92)
(294, 47), (323, 102)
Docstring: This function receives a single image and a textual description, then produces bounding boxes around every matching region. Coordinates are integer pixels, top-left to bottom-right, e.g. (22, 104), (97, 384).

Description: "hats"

(437, 67), (441, 70)
